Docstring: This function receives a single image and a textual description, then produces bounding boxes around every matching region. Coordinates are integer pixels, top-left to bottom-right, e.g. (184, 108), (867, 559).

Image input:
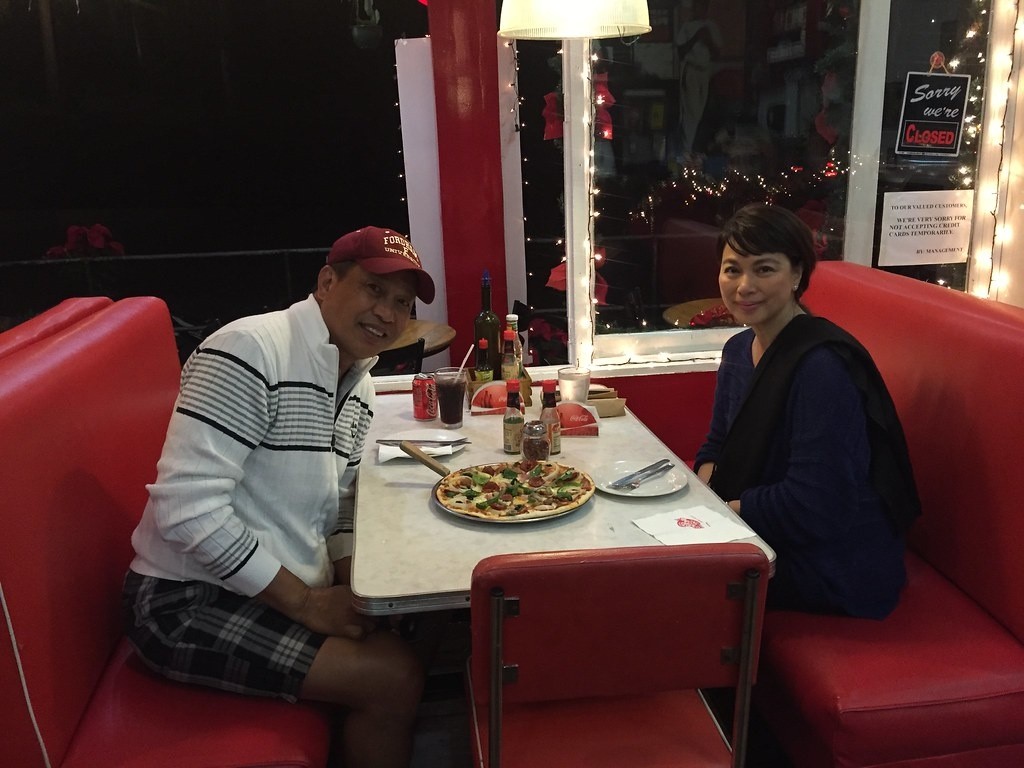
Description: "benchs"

(768, 259), (1023, 768)
(0, 294), (328, 768)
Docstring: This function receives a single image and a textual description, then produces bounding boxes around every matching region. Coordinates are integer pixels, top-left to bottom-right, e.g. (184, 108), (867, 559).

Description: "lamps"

(494, 0), (653, 43)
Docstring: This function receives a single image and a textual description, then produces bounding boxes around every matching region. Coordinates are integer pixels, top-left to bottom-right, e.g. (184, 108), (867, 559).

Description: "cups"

(558, 366), (591, 405)
(434, 366), (468, 429)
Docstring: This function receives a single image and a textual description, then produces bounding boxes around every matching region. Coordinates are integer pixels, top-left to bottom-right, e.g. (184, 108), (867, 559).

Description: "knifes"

(376, 440), (472, 445)
(606, 459), (670, 488)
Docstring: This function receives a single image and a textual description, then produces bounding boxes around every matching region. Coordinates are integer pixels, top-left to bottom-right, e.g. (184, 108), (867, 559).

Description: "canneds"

(413, 372), (440, 422)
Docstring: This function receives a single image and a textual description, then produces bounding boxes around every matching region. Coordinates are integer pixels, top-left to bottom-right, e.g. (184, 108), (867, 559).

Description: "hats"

(329, 225), (436, 306)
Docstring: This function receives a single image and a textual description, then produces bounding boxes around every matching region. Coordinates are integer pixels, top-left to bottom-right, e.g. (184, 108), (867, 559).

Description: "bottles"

(503, 380), (524, 455)
(540, 380), (561, 455)
(474, 278), (522, 380)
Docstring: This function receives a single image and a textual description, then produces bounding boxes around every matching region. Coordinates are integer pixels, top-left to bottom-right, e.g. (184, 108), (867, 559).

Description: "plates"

(379, 429), (466, 458)
(589, 461), (687, 497)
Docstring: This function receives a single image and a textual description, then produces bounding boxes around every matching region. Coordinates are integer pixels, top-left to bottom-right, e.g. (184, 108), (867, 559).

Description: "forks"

(621, 463), (675, 490)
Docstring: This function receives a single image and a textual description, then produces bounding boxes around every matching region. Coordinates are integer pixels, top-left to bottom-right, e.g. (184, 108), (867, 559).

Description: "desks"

(348, 380), (779, 617)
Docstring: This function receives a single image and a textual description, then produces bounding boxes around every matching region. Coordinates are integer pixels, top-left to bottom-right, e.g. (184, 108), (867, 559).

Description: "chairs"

(464, 543), (767, 768)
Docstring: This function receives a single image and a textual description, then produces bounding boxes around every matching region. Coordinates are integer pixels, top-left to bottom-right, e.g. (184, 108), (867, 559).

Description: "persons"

(691, 204), (917, 768)
(119, 224), (423, 768)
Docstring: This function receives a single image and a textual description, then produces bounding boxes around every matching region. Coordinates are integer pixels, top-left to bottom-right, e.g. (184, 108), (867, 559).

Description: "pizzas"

(436, 459), (595, 521)
